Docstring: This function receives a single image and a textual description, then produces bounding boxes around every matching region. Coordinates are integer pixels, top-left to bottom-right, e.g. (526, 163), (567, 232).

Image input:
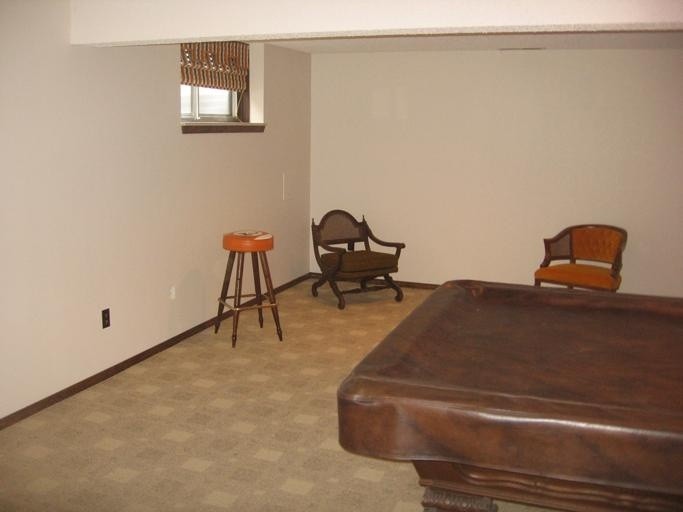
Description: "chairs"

(535, 225), (627, 293)
(309, 208), (407, 308)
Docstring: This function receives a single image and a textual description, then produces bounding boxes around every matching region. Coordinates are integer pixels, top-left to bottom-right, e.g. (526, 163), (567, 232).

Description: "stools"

(215, 231), (283, 346)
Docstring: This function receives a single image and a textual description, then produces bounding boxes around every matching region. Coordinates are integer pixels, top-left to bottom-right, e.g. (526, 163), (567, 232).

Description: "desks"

(334, 280), (682, 512)
